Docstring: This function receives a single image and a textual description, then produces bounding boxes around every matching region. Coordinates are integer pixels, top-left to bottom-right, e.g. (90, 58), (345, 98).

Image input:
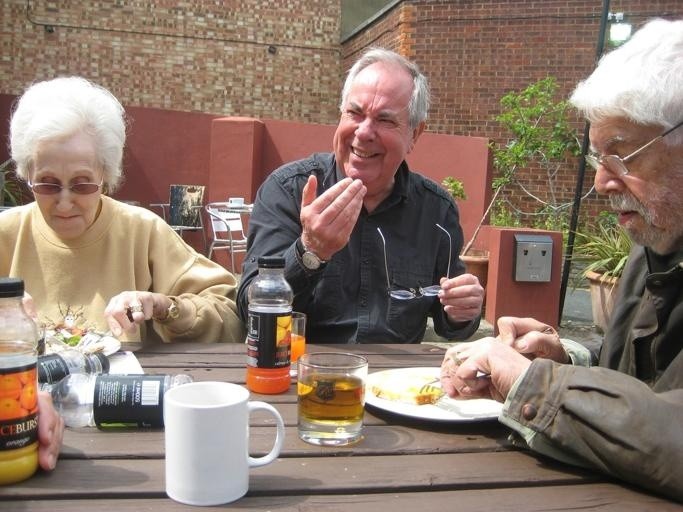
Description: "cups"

(163, 381), (285, 506)
(290, 312), (305, 383)
(297, 351), (369, 446)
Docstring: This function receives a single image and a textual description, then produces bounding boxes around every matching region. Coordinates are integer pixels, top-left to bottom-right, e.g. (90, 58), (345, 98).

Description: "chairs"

(150, 184), (207, 252)
(205, 201), (260, 276)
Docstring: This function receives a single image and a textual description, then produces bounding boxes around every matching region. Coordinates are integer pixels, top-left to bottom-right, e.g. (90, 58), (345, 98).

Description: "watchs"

(153, 298), (180, 322)
(301, 237), (329, 269)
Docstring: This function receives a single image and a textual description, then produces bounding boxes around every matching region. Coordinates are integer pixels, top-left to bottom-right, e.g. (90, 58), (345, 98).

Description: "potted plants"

(444, 78), (592, 307)
(569, 223), (634, 333)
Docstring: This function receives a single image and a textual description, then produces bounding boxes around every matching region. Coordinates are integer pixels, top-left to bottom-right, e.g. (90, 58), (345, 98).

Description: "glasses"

(375, 222), (453, 302)
(583, 120), (681, 179)
(26, 162), (106, 196)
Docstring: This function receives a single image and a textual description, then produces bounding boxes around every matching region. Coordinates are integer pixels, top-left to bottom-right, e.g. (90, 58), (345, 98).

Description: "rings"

(130, 305), (143, 312)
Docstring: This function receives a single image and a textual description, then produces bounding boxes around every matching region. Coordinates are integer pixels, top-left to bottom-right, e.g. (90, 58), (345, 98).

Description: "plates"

(366, 366), (504, 422)
(39, 329), (121, 356)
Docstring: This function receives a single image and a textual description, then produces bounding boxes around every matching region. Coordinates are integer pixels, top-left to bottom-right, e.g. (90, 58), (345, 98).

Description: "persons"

(0, 76), (246, 344)
(236, 47), (485, 344)
(441, 16), (683, 505)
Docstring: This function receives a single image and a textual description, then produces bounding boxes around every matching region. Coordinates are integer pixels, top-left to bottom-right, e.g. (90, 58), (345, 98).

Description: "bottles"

(246, 257), (290, 395)
(36, 350), (110, 389)
(0, 279), (40, 485)
(45, 374), (193, 428)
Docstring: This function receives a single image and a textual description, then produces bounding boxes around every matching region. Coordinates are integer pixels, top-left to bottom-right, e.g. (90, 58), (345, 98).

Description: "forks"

(418, 374), (492, 395)
(75, 325), (112, 351)
